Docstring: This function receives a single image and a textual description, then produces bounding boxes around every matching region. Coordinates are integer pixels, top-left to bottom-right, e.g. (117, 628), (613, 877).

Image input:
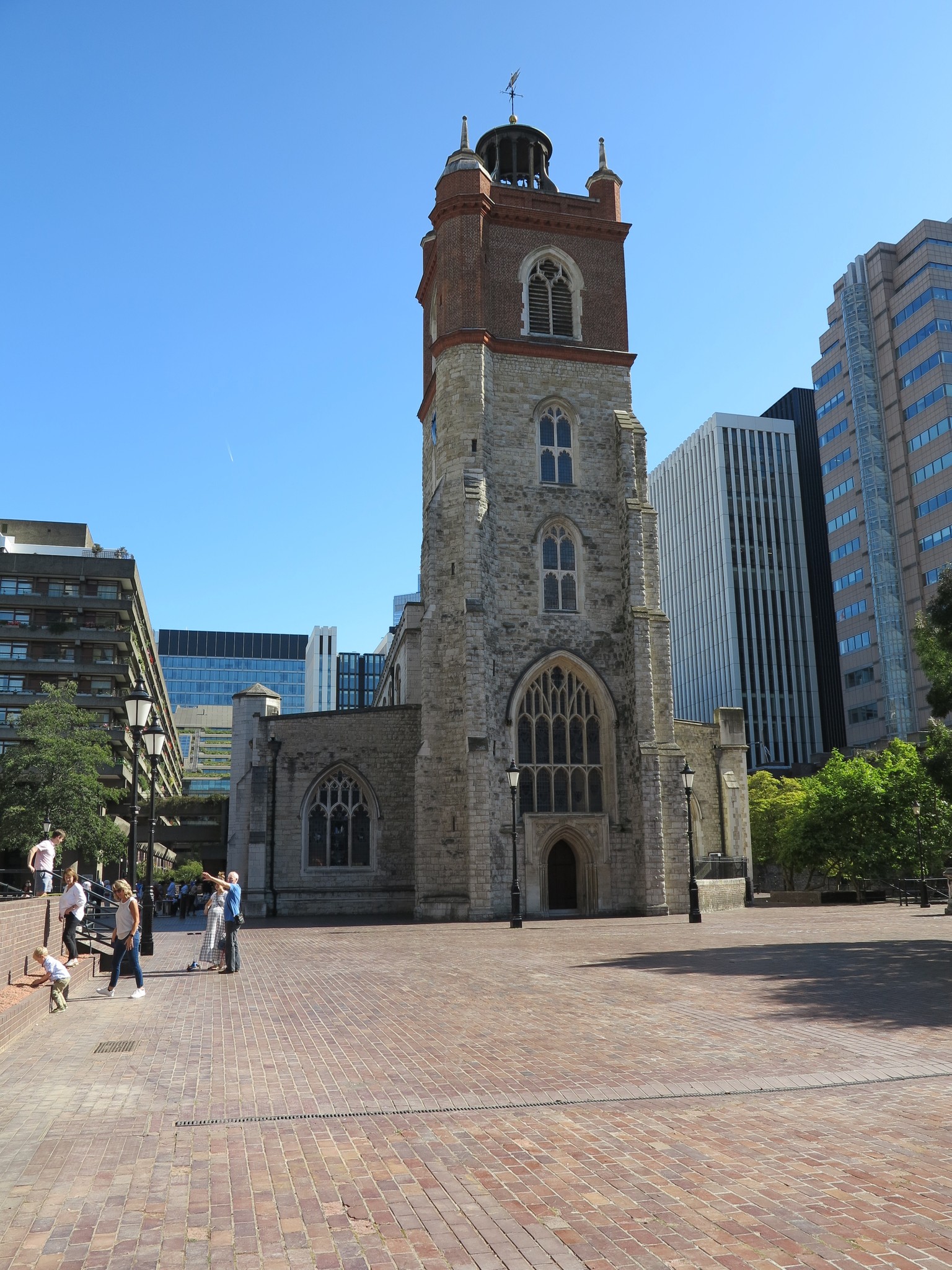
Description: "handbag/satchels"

(234, 913), (245, 925)
(218, 936), (227, 951)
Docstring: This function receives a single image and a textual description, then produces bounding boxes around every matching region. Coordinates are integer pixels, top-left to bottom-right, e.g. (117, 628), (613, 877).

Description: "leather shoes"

(218, 969), (239, 974)
(153, 909), (207, 919)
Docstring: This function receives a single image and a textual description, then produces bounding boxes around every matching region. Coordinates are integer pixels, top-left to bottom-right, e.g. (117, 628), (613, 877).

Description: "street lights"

(679, 760), (702, 924)
(43, 813), (52, 840)
(912, 800), (931, 908)
(117, 672), (157, 976)
(504, 758), (523, 929)
(141, 711), (168, 956)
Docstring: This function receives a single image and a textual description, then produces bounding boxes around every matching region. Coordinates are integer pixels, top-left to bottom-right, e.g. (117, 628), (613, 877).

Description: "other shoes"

(208, 963), (226, 971)
(63, 958), (79, 967)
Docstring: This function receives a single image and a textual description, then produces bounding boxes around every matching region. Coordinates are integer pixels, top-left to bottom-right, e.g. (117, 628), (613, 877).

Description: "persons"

(27, 829), (66, 896)
(135, 877), (214, 920)
(30, 946), (71, 1013)
(16, 879), (34, 897)
(58, 867), (87, 967)
(202, 872), (241, 974)
(199, 871), (228, 971)
(95, 879), (146, 999)
(82, 877), (112, 919)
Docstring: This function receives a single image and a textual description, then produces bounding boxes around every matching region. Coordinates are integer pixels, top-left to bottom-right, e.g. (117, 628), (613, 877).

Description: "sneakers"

(51, 1006), (66, 1013)
(96, 986), (115, 997)
(54, 1004), (69, 1010)
(130, 988), (146, 998)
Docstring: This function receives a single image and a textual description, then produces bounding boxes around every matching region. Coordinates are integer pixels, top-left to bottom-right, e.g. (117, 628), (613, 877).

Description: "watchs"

(73, 905), (76, 909)
(129, 934), (134, 937)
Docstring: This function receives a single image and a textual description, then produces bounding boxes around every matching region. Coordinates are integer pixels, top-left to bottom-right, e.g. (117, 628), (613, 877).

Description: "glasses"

(113, 888), (123, 894)
(214, 882), (218, 886)
